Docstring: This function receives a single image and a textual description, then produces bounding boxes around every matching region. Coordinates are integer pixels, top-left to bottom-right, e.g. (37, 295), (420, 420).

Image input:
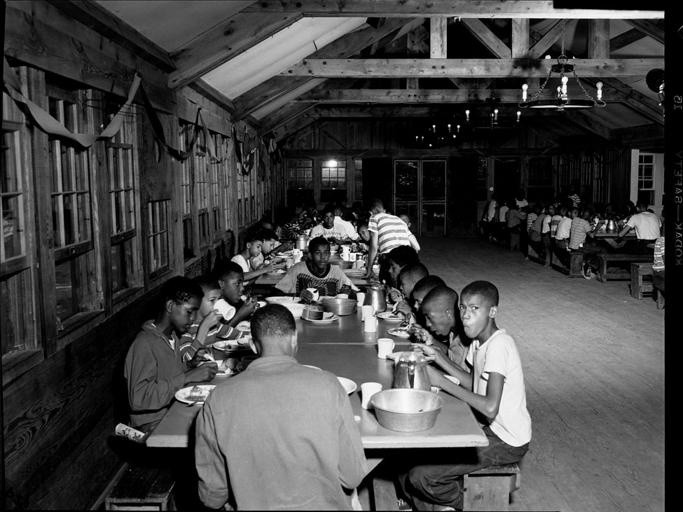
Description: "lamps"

(515, 19), (608, 113)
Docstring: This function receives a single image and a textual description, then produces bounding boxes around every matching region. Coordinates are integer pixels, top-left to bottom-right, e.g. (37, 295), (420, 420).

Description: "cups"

(363, 315), (379, 332)
(360, 382), (383, 408)
(361, 305), (373, 320)
(213, 299), (236, 321)
(377, 338), (395, 359)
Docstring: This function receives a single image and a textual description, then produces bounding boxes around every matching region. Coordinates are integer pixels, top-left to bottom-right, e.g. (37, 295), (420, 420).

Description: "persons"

(480, 189), (665, 311)
(176, 277), (250, 368)
(120, 273), (218, 432)
(195, 304), (369, 509)
(217, 197), (472, 381)
(395, 281), (534, 512)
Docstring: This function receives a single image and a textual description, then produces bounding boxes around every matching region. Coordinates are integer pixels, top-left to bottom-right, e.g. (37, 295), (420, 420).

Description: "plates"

(388, 327), (430, 339)
(198, 359), (235, 377)
(213, 338), (258, 353)
(281, 303), (306, 319)
(266, 296), (301, 303)
(388, 351), (437, 362)
(584, 214), (628, 232)
(376, 311), (414, 323)
(303, 312), (340, 325)
(174, 384), (217, 406)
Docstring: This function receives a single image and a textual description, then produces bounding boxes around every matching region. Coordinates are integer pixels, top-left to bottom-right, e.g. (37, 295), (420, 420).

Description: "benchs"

(554, 239), (584, 279)
(461, 463), (521, 512)
(104, 459), (180, 512)
(596, 252), (656, 300)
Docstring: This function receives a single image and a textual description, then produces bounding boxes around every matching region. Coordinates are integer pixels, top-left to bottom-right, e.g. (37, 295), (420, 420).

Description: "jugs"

(393, 353), (436, 390)
(363, 281), (387, 314)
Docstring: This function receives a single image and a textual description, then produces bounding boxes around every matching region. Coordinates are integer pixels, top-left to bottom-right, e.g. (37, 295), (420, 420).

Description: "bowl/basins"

(370, 388), (444, 432)
(322, 297), (358, 315)
